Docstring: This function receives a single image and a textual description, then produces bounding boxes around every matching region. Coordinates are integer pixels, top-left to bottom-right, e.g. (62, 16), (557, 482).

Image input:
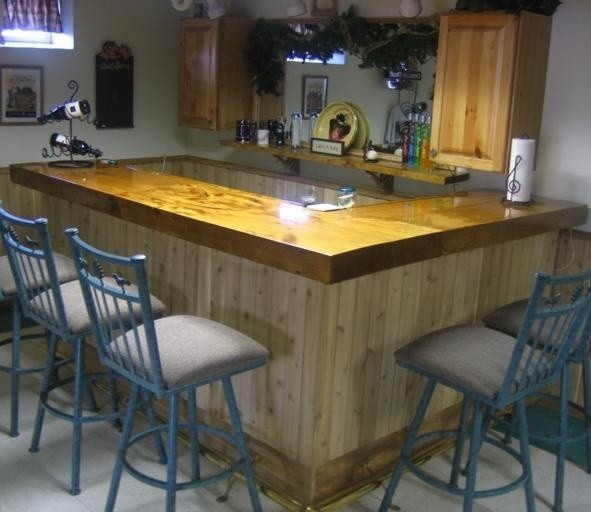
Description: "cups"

(236, 119), (257, 143)
(255, 129), (270, 145)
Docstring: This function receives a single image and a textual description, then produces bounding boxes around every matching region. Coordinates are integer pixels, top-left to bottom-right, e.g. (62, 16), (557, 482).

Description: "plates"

(314, 101), (357, 149)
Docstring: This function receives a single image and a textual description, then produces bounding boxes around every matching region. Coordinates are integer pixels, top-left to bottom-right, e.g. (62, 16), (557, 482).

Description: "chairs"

(462, 295), (590, 512)
(0, 246), (100, 437)
(382, 270), (590, 512)
(63, 226), (276, 511)
(1, 202), (168, 495)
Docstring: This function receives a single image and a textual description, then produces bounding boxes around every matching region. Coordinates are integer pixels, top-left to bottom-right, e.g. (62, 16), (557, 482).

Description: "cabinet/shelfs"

(427, 9), (553, 174)
(179, 17), (283, 132)
(218, 14), (471, 194)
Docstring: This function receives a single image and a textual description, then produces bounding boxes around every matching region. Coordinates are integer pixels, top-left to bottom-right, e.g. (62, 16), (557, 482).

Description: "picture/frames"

(301, 74), (328, 119)
(0, 64), (46, 125)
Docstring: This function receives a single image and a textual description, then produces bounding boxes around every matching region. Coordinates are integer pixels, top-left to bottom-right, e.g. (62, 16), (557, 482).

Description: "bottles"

(50, 131), (102, 159)
(404, 110), (431, 163)
(292, 110), (302, 149)
(38, 99), (90, 121)
